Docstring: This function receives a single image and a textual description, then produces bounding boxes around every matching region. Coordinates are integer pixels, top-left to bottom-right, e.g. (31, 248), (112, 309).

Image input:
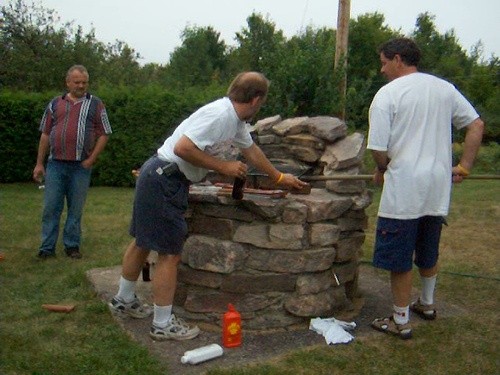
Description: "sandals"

(410, 297), (436, 321)
(371, 314), (412, 339)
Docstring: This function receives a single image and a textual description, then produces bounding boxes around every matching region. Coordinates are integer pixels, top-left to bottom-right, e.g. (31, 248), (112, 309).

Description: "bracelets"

(274, 173), (284, 190)
(457, 164), (469, 176)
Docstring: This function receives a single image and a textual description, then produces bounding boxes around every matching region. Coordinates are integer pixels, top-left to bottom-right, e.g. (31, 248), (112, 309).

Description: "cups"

(36, 173), (45, 189)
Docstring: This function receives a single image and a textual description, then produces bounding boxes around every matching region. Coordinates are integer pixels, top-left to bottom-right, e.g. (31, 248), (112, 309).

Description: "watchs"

(378, 164), (386, 173)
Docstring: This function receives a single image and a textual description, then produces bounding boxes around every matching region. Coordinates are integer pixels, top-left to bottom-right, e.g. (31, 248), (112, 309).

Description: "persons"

(108, 71), (308, 341)
(366, 39), (484, 339)
(27, 65), (113, 261)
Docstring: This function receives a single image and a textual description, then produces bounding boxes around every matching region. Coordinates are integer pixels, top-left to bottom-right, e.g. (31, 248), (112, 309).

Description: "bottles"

(232, 156), (249, 202)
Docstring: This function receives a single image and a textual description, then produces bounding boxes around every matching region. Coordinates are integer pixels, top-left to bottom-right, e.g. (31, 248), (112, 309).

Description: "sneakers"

(149, 314), (200, 341)
(108, 294), (152, 319)
(35, 251), (55, 261)
(65, 246), (81, 258)
